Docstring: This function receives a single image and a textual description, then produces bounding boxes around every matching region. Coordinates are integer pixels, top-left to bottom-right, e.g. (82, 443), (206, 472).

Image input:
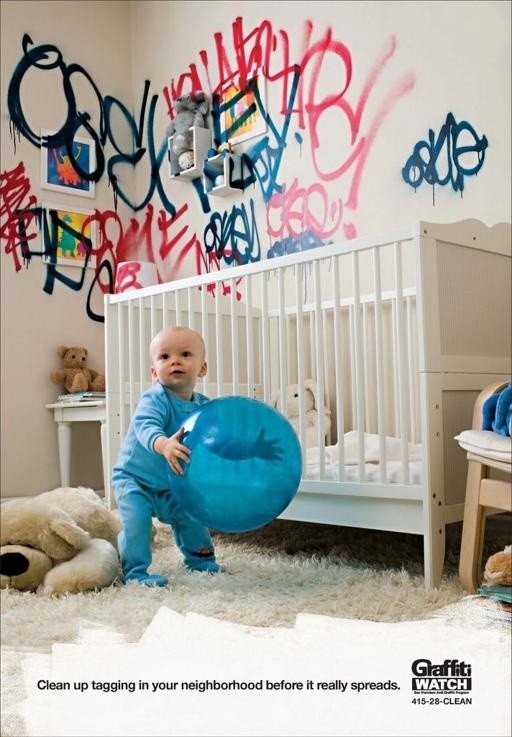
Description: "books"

(57, 392), (105, 402)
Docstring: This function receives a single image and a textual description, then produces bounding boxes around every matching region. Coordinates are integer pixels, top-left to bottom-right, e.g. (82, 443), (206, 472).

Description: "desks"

(45, 401), (110, 511)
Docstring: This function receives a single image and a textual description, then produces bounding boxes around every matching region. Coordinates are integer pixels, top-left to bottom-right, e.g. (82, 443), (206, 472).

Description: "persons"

(484, 545), (512, 586)
(481, 381), (512, 437)
(0, 485), (157, 595)
(166, 91), (209, 176)
(270, 379), (331, 451)
(112, 326), (285, 588)
(50, 345), (105, 392)
(167, 396), (303, 532)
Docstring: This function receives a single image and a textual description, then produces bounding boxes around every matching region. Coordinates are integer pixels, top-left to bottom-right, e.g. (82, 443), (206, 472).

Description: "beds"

(103, 218), (512, 589)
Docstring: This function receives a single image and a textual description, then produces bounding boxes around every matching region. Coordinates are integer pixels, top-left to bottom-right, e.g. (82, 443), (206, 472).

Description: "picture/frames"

(41, 201), (97, 270)
(219, 74), (268, 146)
(40, 128), (96, 199)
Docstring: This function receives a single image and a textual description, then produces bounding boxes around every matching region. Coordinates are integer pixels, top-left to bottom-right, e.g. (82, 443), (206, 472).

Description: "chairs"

(453, 380), (512, 594)
